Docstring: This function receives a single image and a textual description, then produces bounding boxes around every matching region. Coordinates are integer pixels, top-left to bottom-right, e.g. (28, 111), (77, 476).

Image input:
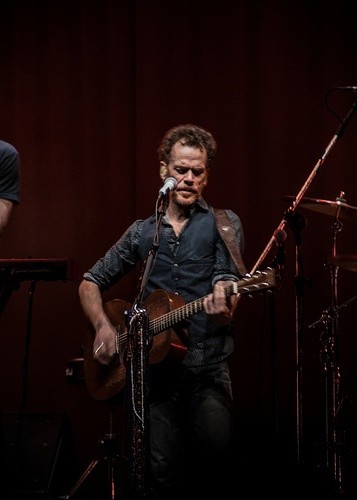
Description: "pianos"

(0, 258), (68, 315)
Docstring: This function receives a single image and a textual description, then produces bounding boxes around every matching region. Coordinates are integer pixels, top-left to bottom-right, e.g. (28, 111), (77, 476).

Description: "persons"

(78, 124), (245, 499)
(0, 139), (20, 230)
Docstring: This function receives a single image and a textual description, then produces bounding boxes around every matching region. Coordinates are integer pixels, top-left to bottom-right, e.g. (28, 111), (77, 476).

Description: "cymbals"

(329, 255), (357, 272)
(288, 196), (357, 220)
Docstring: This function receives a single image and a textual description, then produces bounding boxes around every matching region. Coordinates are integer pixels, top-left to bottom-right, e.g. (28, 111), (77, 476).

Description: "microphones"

(158, 176), (178, 197)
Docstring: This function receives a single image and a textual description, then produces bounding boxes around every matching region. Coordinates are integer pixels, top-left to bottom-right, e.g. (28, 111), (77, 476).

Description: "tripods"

(60, 401), (134, 500)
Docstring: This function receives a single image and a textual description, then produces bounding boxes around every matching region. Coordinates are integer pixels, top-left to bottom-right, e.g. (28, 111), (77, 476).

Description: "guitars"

(82, 266), (275, 401)
